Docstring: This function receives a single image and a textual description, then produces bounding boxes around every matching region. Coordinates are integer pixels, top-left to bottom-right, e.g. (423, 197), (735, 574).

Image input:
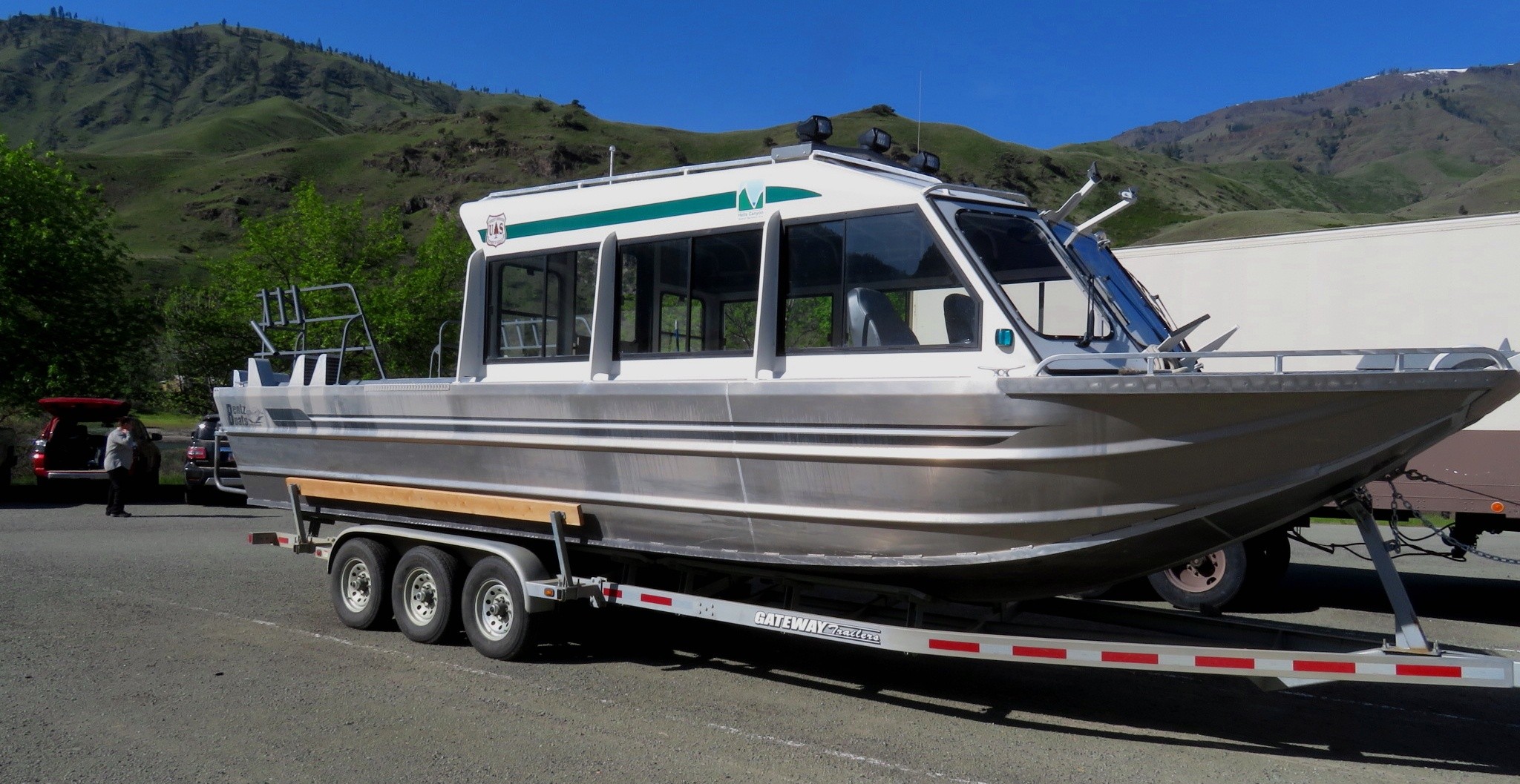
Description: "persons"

(103, 416), (133, 518)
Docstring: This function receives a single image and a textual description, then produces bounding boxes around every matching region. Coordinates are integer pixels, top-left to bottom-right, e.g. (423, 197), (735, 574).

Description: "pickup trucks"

(0, 426), (17, 504)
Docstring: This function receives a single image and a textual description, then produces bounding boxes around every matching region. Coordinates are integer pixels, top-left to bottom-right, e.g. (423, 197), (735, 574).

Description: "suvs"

(185, 412), (250, 506)
(28, 397), (162, 504)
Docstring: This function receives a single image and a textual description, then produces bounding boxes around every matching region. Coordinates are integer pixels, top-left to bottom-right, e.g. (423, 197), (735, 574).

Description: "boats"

(210, 68), (1520, 596)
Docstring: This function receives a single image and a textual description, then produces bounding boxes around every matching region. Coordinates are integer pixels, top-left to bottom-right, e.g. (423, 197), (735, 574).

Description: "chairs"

(944, 293), (978, 341)
(72, 422), (90, 436)
(846, 287), (921, 346)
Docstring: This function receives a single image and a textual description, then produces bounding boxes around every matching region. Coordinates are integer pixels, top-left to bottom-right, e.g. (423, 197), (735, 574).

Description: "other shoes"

(106, 511), (132, 517)
